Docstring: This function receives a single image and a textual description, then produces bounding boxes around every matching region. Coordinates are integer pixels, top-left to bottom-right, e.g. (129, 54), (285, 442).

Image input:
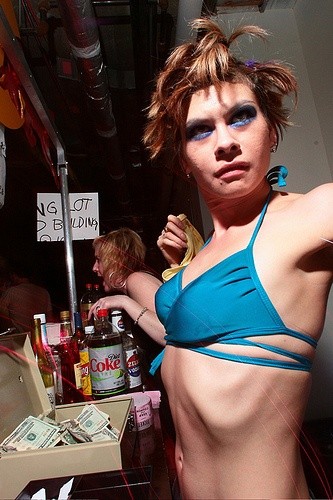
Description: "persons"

(0, 262), (55, 355)
(142, 15), (333, 499)
(86, 227), (168, 346)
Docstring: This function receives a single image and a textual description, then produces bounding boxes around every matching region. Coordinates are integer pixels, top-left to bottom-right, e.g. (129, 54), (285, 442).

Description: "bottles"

(87, 309), (130, 401)
(30, 318), (56, 407)
(59, 311), (76, 404)
(111, 307), (142, 394)
(34, 314), (59, 408)
(80, 325), (94, 404)
(80, 284), (96, 332)
(92, 284), (102, 302)
(69, 311), (85, 403)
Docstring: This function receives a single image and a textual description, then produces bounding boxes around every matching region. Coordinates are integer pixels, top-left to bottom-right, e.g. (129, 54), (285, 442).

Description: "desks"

(21, 370), (173, 500)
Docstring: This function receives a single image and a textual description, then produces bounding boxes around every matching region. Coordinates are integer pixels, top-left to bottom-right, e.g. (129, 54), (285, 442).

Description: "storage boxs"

(0, 329), (139, 500)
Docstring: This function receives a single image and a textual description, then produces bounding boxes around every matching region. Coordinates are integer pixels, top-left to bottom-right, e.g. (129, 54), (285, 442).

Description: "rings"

(164, 227), (167, 232)
(96, 306), (100, 310)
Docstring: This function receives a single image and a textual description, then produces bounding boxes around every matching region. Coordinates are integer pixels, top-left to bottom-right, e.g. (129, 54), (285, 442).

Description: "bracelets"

(134, 307), (148, 325)
